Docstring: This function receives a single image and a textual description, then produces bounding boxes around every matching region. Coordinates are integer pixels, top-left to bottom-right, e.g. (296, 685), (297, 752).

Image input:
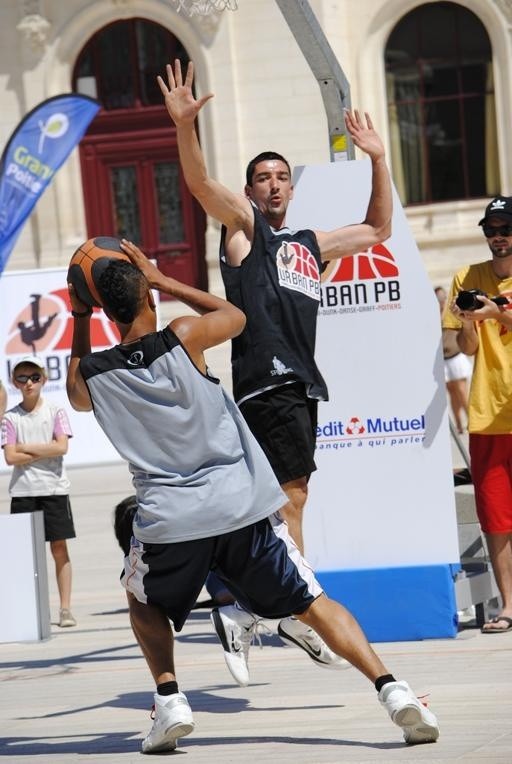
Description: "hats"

(478, 197), (512, 226)
(12, 354), (46, 371)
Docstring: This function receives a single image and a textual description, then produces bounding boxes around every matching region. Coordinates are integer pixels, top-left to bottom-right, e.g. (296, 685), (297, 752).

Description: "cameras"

(456, 289), (509, 310)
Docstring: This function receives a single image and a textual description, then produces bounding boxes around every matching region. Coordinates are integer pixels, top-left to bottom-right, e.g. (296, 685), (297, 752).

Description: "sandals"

(481, 616), (512, 633)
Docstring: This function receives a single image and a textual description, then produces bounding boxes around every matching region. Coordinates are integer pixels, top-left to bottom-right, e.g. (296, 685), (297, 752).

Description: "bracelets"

(71, 307), (95, 319)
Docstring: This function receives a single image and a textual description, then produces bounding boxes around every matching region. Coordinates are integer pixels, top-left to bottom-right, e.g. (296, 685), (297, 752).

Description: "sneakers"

(277, 616), (355, 671)
(209, 605), (263, 687)
(378, 680), (440, 744)
(59, 608), (76, 627)
(141, 691), (194, 753)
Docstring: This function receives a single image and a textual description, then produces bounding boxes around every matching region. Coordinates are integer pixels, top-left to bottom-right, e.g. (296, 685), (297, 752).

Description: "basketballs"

(68, 237), (132, 308)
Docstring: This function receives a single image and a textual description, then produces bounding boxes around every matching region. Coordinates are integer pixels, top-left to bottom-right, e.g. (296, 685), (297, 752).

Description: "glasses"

(14, 373), (44, 384)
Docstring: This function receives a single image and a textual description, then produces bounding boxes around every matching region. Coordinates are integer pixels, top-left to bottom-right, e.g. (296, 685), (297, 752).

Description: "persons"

(434, 285), (471, 435)
(440, 193), (512, 633)
(65, 234), (440, 753)
(2, 356), (78, 630)
(156, 52), (394, 688)
